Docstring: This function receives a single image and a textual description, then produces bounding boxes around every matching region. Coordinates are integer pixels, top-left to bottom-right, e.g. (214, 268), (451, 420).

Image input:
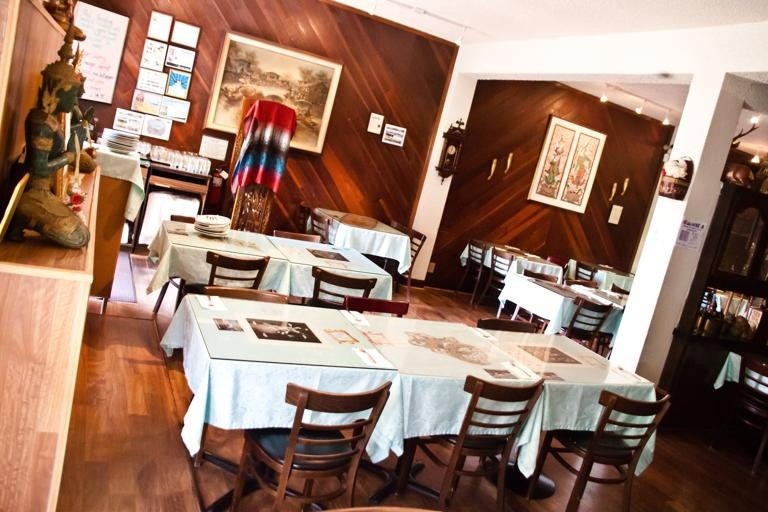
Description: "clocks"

(436, 118), (467, 184)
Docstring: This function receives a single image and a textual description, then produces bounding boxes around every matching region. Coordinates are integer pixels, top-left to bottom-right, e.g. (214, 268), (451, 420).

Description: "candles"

(612, 183), (618, 196)
(507, 152), (513, 169)
(491, 158), (498, 174)
(623, 178), (630, 192)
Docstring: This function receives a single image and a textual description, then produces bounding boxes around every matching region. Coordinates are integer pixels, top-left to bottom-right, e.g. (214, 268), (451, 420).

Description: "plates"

(104, 129), (139, 154)
(194, 214), (232, 239)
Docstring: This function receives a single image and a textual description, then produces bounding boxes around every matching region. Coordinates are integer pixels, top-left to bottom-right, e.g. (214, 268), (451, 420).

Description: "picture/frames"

(202, 29), (345, 156)
(526, 116), (608, 216)
(145, 10), (202, 99)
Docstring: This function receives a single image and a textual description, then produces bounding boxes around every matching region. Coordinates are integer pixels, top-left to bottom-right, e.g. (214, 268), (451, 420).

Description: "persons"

(545, 139), (564, 186)
(573, 142), (591, 187)
(10, 12), (91, 248)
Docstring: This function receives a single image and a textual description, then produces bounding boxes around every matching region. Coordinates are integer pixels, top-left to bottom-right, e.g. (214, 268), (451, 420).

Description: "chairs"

(672, 286), (766, 479)
(153, 207), (670, 510)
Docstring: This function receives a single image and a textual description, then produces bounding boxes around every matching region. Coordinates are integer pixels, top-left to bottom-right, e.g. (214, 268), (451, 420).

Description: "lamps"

(600, 83), (673, 126)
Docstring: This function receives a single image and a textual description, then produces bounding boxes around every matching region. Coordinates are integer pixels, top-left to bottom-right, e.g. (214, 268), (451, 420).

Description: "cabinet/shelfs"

(90, 151), (139, 317)
(0, 166), (102, 512)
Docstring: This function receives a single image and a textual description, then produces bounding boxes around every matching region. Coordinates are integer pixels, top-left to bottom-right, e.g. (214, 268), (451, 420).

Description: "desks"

(131, 161), (213, 253)
